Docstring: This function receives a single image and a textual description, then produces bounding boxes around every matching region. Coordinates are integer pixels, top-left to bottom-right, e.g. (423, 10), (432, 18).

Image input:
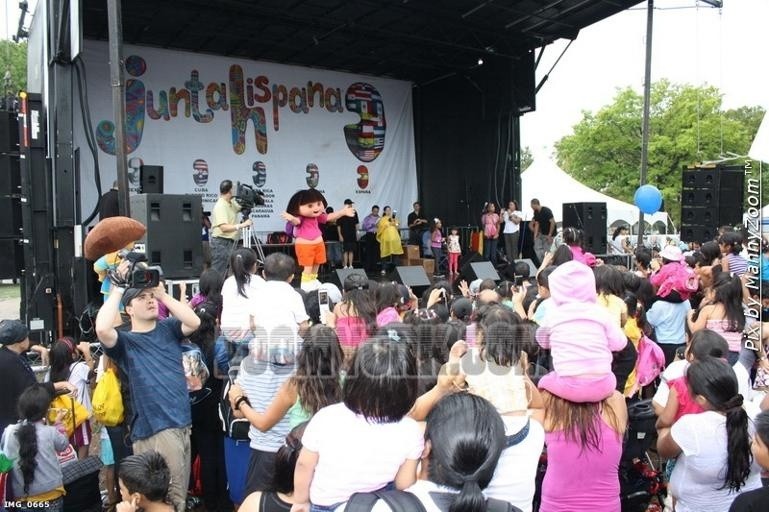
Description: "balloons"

(635, 184), (664, 216)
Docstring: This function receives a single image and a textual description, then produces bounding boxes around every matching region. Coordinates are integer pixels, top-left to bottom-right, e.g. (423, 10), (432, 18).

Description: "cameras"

(115, 252), (159, 289)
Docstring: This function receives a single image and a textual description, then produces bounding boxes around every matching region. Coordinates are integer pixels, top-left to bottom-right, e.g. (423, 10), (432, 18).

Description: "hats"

(658, 245), (686, 261)
(344, 199), (353, 204)
(0, 319), (30, 345)
(122, 285), (144, 306)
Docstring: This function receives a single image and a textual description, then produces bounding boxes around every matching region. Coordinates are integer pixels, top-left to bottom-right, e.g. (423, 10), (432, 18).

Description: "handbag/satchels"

(92, 357), (125, 428)
(50, 395), (88, 438)
(0, 453), (13, 508)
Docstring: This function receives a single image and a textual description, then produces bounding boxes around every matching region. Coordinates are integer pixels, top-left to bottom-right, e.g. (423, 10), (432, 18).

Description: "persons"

(481, 201), (502, 268)
(277, 188), (357, 293)
(335, 198), (359, 270)
(529, 198), (557, 265)
(407, 201), (431, 259)
(97, 179), (120, 223)
(361, 204), (383, 272)
(375, 205), (405, 276)
(199, 207), (211, 242)
(498, 199), (525, 263)
(1, 215), (769, 511)
(204, 178), (253, 286)
(322, 206), (347, 273)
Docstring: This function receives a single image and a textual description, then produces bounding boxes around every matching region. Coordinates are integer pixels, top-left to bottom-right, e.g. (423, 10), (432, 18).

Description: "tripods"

(221, 216), (265, 280)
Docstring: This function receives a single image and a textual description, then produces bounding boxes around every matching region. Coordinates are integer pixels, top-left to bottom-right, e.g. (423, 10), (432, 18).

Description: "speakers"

(680, 164), (744, 244)
(562, 202), (607, 254)
(511, 258), (538, 279)
(129, 193), (203, 279)
(140, 165), (164, 193)
(388, 265), (431, 298)
(329, 268), (368, 290)
(452, 261), (501, 294)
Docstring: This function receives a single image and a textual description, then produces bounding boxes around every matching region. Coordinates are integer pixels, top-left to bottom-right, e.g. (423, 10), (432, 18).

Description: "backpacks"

(181, 343), (213, 408)
(629, 335), (666, 398)
(216, 345), (252, 443)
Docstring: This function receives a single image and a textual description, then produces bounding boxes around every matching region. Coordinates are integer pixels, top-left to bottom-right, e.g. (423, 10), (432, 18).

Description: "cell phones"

(317, 289), (331, 326)
(514, 275), (524, 294)
